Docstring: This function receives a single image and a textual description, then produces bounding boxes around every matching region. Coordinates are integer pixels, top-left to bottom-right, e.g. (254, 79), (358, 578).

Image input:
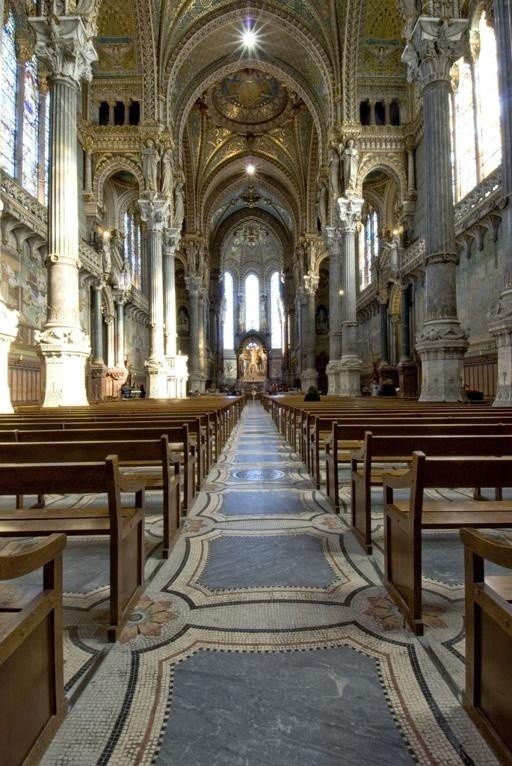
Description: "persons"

(141, 139), (161, 192)
(304, 385), (321, 401)
(381, 379), (397, 396)
(341, 139), (359, 190)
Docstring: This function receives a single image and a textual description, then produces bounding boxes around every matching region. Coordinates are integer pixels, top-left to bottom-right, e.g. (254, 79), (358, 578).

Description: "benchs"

(264, 396), (507, 764)
(0, 395), (247, 765)
